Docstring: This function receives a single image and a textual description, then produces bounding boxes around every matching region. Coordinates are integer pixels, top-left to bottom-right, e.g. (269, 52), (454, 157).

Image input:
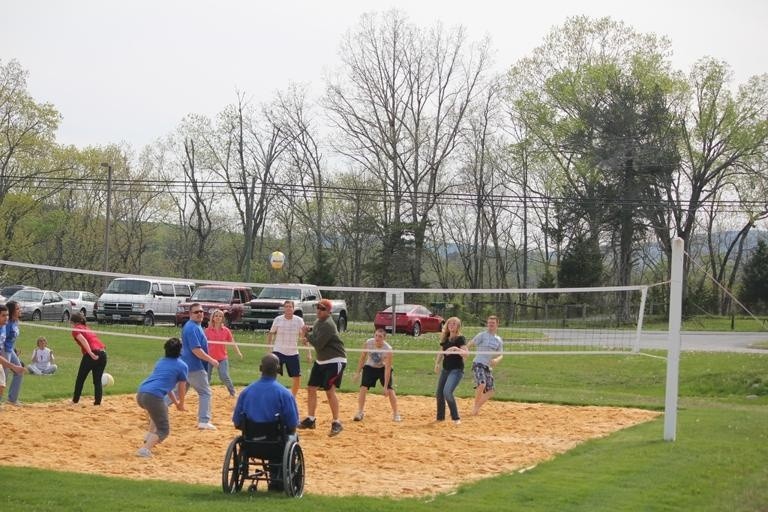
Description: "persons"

(232, 353), (300, 491)
(70, 313), (107, 408)
(135, 337), (189, 457)
(468, 315), (504, 415)
(166, 303), (220, 430)
(297, 298), (347, 436)
(204, 309), (243, 398)
(1, 300), (58, 406)
(268, 300), (312, 398)
(433, 317), (469, 425)
(353, 327), (400, 422)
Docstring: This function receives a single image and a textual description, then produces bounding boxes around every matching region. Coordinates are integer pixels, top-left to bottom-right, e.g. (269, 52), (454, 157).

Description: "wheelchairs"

(220, 412), (307, 497)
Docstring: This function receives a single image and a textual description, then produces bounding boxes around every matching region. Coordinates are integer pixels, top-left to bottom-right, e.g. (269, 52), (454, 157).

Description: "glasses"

(316, 305), (326, 311)
(190, 310), (203, 315)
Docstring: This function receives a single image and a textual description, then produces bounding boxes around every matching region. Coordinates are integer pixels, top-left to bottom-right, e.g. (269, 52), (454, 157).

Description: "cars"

(374, 305), (449, 336)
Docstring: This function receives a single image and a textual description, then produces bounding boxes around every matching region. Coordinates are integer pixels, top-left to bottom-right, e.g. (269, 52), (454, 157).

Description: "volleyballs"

(269, 251), (286, 268)
(101, 373), (114, 387)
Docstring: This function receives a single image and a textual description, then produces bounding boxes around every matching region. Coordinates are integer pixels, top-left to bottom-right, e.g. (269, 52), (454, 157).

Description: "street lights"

(98, 160), (111, 297)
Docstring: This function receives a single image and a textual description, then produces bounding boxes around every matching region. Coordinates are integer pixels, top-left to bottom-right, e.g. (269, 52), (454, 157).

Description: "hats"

(315, 298), (332, 310)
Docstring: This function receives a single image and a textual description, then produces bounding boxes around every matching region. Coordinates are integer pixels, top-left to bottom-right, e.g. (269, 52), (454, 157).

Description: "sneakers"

(297, 418), (316, 430)
(433, 418), (462, 425)
(198, 421), (218, 430)
(136, 431), (153, 458)
(328, 421), (344, 439)
(354, 412), (403, 422)
